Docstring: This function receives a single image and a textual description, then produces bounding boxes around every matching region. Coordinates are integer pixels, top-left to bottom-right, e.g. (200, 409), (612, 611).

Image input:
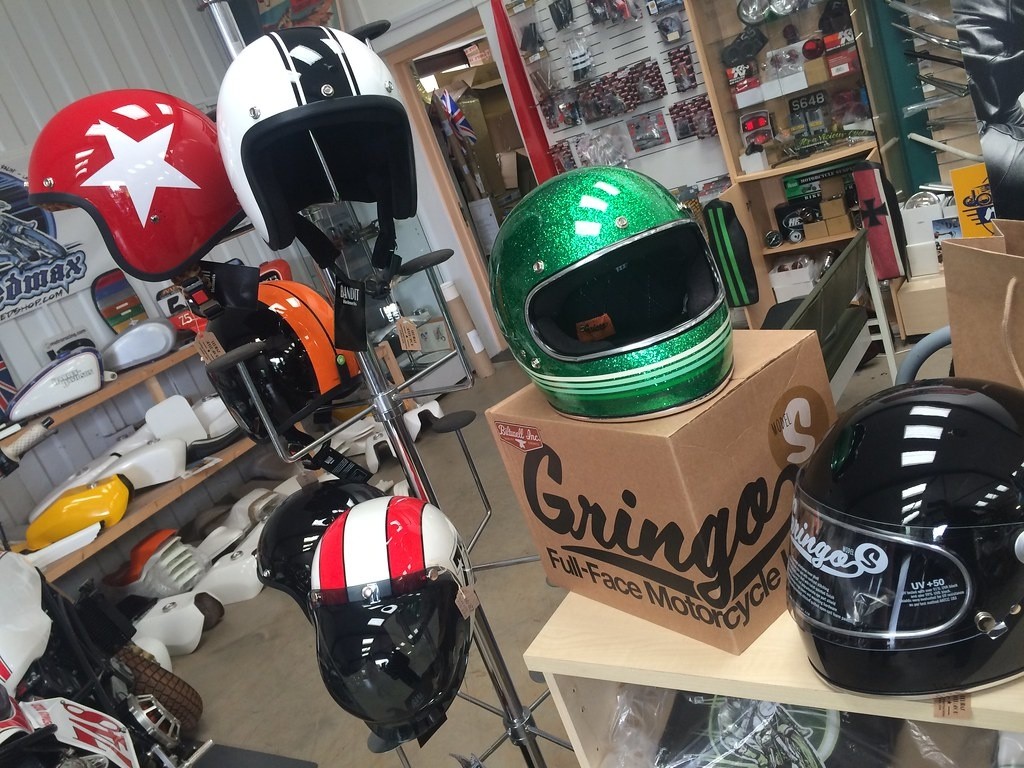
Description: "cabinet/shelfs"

(308, 166), (475, 387)
(522, 594), (1023, 768)
(0, 336), (322, 628)
(683, 0), (911, 340)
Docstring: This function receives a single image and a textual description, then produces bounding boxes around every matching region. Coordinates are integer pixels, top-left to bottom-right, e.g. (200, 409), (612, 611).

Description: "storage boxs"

(481, 329), (843, 671)
(433, 36), (537, 206)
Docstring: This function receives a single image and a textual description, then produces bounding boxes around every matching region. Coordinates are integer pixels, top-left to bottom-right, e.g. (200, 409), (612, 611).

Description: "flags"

(428, 88), (478, 146)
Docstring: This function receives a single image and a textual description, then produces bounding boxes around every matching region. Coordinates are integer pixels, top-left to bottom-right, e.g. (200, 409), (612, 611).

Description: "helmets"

(29, 89), (246, 281)
(308, 494), (475, 744)
(786, 376), (1024, 699)
(256, 478), (387, 633)
(217, 25), (419, 249)
(203, 278), (361, 405)
(487, 164), (734, 424)
